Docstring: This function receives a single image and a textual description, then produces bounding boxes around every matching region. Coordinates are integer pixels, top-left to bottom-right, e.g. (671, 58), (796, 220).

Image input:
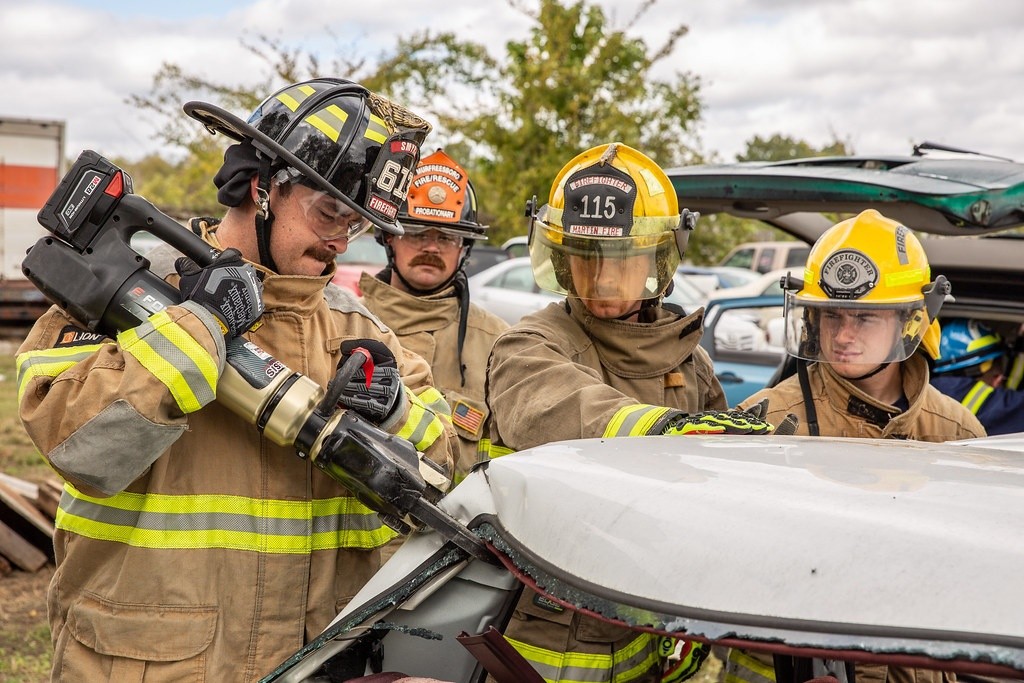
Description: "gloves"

(325, 338), (400, 424)
(174, 247), (267, 357)
(660, 398), (775, 434)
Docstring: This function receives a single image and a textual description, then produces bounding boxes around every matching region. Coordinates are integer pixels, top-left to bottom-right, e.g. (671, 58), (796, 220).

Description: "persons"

(735, 208), (987, 683)
(469, 142), (774, 683)
(16, 79), (459, 682)
(358, 148), (514, 565)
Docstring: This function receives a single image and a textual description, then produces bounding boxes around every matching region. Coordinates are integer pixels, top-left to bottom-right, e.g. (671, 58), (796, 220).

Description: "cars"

(328, 229), (813, 421)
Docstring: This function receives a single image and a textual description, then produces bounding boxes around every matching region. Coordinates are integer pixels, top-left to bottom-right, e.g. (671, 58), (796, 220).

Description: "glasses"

(399, 231), (463, 253)
(294, 172), (373, 242)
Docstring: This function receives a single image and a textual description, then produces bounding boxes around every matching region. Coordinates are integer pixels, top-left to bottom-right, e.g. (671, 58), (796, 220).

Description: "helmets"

(534, 142), (679, 258)
(786, 209), (931, 310)
(932, 319), (1006, 374)
(183, 76), (432, 236)
(374, 148), (489, 234)
